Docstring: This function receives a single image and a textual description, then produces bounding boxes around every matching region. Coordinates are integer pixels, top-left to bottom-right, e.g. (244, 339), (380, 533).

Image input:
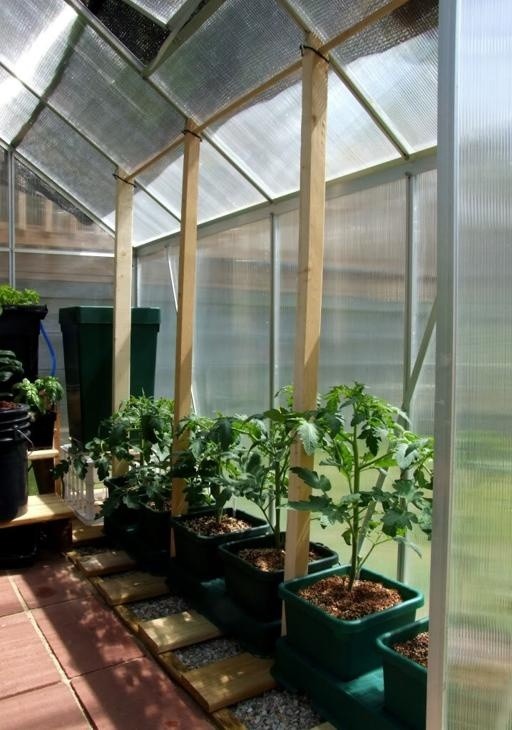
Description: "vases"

(371, 614), (428, 730)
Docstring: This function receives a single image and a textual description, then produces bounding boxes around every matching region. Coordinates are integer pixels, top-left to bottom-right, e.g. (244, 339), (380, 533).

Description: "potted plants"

(278, 383), (435, 677)
(170, 412), (271, 583)
(45, 384), (219, 567)
(2, 283), (64, 506)
(222, 405), (339, 619)
